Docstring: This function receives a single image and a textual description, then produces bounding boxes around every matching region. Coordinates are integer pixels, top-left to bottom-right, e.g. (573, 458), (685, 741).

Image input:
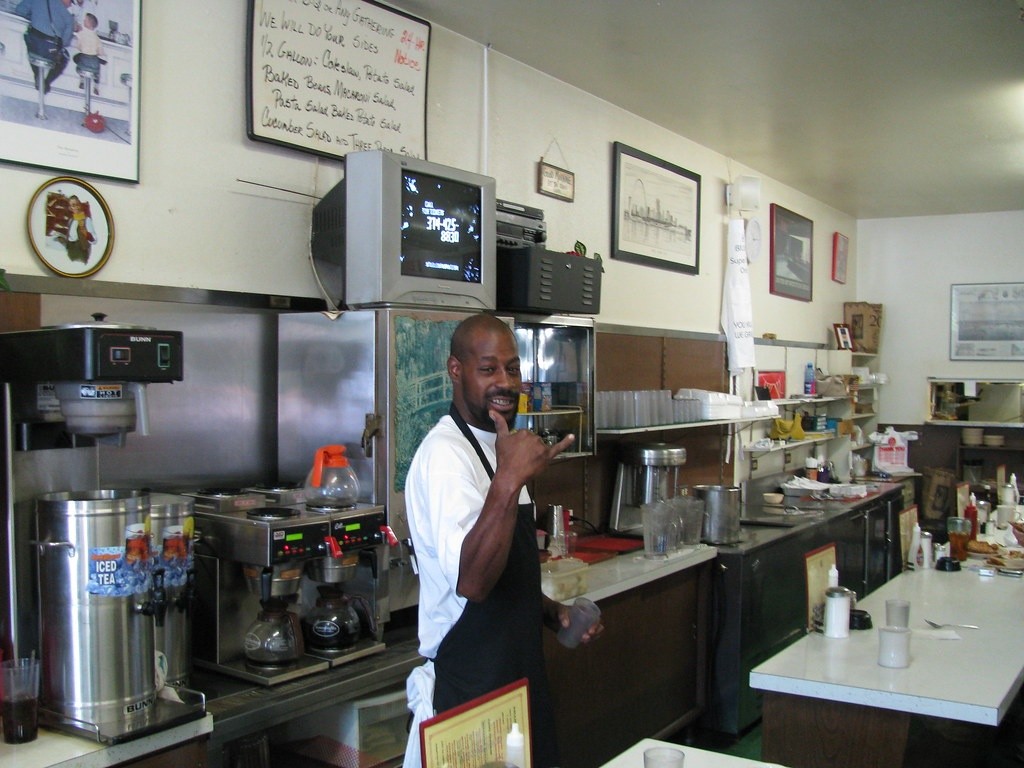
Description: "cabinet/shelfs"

(0, 382), (1024, 768)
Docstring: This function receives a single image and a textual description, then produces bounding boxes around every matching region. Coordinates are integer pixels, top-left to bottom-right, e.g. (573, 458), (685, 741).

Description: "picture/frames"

(610, 141), (702, 276)
(832, 232), (848, 283)
(27, 177), (115, 278)
(769, 203), (814, 302)
(0, 0), (143, 185)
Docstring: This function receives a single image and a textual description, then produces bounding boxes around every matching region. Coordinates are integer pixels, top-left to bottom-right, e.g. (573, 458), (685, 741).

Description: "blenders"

(1, 313), (207, 746)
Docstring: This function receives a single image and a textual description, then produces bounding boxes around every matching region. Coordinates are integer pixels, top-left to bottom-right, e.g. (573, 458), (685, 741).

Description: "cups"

(997, 505), (1022, 522)
(595, 389), (702, 429)
(556, 597), (600, 648)
(878, 626), (911, 668)
(885, 598), (910, 627)
(806, 467), (817, 480)
(640, 484), (742, 557)
(643, 747), (685, 768)
(947, 517), (971, 562)
(817, 471), (830, 483)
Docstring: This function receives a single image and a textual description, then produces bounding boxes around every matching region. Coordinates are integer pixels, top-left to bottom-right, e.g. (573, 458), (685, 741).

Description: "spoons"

(924, 618), (979, 630)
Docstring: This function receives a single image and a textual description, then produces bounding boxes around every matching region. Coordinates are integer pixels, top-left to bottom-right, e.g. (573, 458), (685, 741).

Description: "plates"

(962, 428), (1005, 446)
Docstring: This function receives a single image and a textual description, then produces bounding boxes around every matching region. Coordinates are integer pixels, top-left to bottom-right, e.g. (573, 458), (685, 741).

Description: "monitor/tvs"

(311, 151), (497, 313)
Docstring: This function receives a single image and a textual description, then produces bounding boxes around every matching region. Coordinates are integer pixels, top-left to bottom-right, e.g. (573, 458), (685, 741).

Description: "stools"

(27, 53), (55, 117)
(75, 65), (97, 126)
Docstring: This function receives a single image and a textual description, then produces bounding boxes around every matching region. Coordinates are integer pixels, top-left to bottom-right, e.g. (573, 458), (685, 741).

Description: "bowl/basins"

(540, 558), (591, 600)
(763, 493), (785, 504)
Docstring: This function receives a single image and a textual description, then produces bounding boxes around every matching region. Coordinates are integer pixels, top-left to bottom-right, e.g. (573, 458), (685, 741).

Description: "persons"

(14, 0), (107, 95)
(403, 314), (604, 768)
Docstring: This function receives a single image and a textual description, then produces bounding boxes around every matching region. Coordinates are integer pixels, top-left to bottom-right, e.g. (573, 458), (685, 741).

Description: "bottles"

(969, 492), (976, 506)
(1001, 484), (1015, 505)
(804, 363), (816, 394)
(920, 531), (933, 569)
(1010, 473), (1020, 504)
(824, 587), (851, 639)
(908, 523), (924, 571)
(963, 500), (978, 541)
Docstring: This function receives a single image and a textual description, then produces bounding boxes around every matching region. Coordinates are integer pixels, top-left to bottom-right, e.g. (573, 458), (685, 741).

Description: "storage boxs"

(280, 687), (413, 768)
(540, 560), (590, 601)
(921, 465), (952, 519)
(845, 301), (883, 354)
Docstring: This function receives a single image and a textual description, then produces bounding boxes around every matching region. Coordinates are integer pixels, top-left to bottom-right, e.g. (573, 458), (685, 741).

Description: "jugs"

(303, 584), (377, 651)
(242, 598), (305, 666)
(303, 446), (361, 508)
